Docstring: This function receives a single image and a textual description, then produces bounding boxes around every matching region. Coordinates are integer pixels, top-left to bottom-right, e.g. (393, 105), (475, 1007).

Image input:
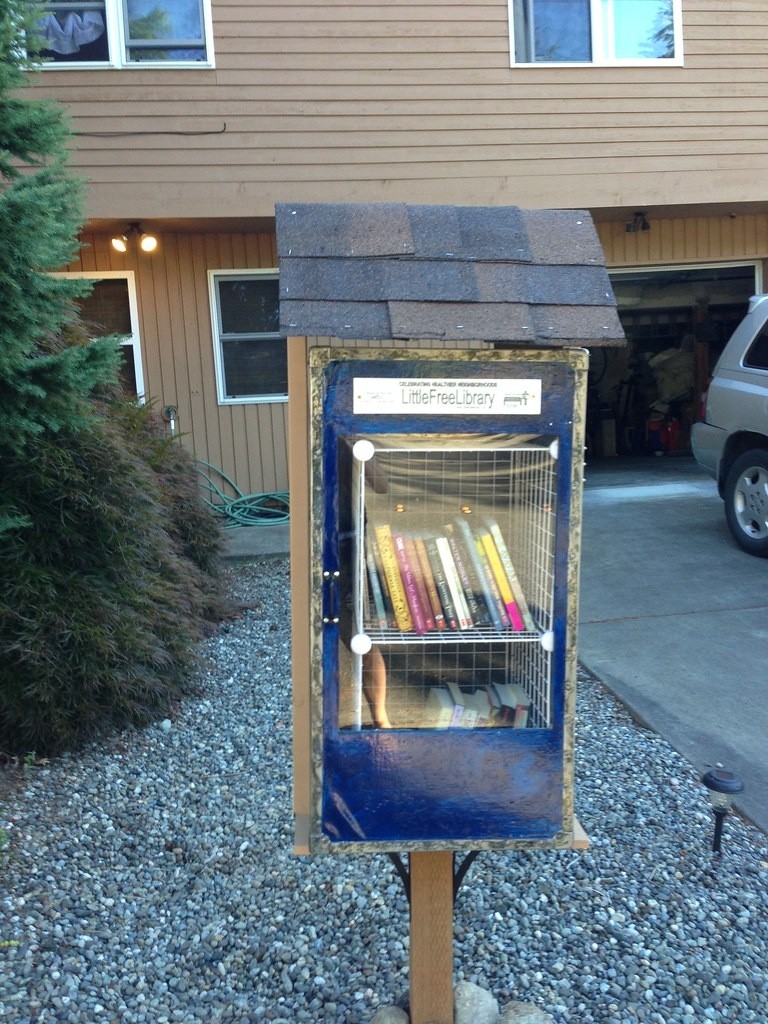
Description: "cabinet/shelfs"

(351, 431), (560, 730)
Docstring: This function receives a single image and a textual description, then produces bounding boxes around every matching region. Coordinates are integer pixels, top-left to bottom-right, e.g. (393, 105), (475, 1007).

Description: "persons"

(322, 426), (388, 730)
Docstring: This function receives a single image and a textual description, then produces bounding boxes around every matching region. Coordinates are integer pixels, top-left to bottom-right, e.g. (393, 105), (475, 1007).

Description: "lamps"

(623, 211), (650, 231)
(112, 222), (157, 252)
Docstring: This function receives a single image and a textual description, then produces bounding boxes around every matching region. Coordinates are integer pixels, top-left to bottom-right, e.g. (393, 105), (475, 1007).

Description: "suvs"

(689, 293), (767, 560)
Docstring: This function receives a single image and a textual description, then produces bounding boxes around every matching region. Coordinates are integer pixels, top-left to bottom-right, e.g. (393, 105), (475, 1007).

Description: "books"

(362, 510), (536, 633)
